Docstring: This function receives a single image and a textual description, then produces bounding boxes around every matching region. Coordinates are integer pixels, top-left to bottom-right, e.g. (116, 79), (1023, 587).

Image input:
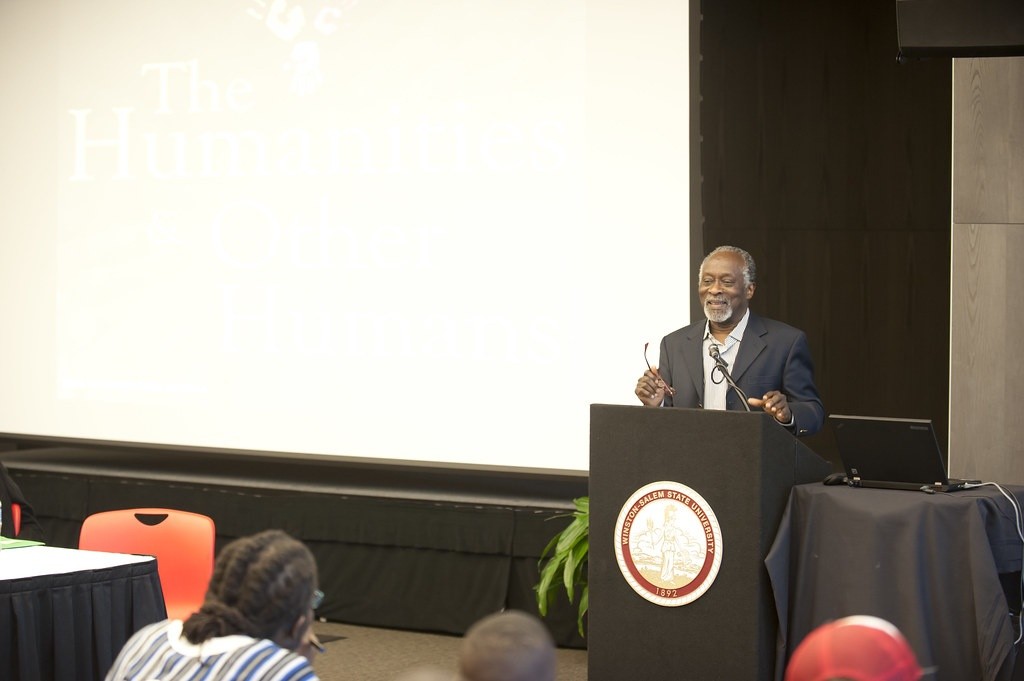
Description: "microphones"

(708, 344), (719, 358)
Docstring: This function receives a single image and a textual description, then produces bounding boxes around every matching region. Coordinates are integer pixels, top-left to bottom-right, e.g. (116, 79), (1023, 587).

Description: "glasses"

(644, 343), (678, 398)
(285, 589), (326, 639)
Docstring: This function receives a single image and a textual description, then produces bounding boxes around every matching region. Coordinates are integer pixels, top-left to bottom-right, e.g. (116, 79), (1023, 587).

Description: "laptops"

(828, 415), (982, 492)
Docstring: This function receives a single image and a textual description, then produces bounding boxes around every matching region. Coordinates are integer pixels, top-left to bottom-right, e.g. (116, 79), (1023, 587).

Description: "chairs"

(78, 506), (217, 623)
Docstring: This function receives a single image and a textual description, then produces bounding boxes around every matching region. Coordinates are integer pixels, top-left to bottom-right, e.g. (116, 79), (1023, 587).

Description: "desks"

(0, 536), (170, 681)
(764, 482), (1024, 681)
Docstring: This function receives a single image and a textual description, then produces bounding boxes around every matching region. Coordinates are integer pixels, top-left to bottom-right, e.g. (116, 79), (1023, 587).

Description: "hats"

(783, 617), (937, 681)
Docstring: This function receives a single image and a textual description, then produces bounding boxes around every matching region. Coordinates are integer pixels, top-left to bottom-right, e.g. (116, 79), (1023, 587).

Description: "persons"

(104, 529), (320, 681)
(458, 609), (558, 681)
(784, 616), (923, 681)
(0, 462), (45, 543)
(635, 245), (829, 438)
